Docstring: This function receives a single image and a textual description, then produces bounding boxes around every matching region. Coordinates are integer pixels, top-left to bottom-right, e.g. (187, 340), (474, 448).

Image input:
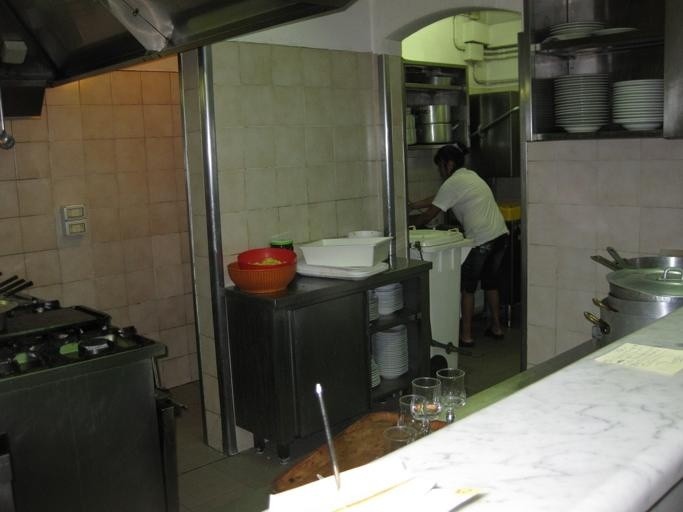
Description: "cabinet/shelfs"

(524, 0), (683, 143)
(402, 61), (470, 151)
(225, 258), (432, 466)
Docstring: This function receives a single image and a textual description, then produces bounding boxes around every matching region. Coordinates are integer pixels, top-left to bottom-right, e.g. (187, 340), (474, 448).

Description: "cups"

(384, 396), (427, 457)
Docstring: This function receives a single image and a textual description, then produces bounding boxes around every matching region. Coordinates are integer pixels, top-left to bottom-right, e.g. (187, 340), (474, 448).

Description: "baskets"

(226, 260), (297, 295)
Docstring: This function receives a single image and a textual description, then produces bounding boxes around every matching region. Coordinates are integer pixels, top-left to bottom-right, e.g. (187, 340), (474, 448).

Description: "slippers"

(459, 327), (505, 348)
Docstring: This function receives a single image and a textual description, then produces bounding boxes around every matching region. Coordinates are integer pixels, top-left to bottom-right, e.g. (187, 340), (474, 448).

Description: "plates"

(368, 284), (410, 392)
(547, 22), (636, 39)
(549, 74), (667, 135)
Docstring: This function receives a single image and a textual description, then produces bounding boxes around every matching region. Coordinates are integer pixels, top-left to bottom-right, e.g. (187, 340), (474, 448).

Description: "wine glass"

(411, 369), (469, 439)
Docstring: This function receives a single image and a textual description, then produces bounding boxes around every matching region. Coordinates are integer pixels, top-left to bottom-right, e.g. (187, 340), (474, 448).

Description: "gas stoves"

(0, 285), (62, 318)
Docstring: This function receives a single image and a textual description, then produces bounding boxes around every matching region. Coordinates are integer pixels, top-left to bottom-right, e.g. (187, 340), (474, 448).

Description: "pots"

(584, 245), (683, 353)
(412, 103), (458, 144)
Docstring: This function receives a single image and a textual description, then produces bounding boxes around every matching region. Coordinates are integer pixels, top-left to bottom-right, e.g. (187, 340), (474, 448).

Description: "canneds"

(271, 240), (293, 251)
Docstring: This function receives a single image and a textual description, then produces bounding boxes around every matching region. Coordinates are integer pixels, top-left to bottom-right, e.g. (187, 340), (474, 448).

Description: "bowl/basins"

(238, 249), (298, 271)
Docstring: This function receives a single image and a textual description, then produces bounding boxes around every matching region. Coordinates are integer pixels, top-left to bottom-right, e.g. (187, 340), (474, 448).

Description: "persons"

(407, 140), (512, 347)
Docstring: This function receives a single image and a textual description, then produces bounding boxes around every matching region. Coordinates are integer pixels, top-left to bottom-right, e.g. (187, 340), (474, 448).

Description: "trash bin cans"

(408, 225), (476, 368)
